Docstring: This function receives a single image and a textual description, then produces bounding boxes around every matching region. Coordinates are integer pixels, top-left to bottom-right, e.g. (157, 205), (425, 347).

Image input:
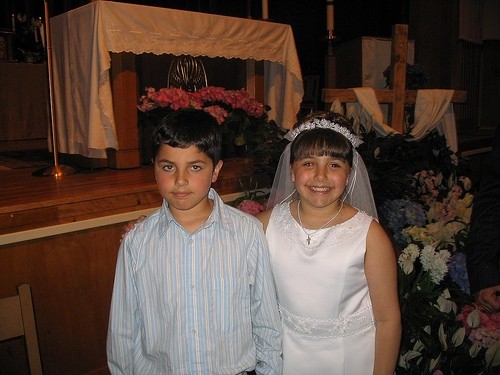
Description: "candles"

(262, 0), (268, 19)
(11, 14), (16, 31)
(40, 23), (46, 48)
(326, 4), (335, 30)
(35, 30), (38, 42)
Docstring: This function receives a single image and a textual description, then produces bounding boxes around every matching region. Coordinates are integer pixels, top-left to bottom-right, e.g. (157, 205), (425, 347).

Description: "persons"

(106, 110), (284, 375)
(466, 126), (499, 313)
(119, 111), (402, 375)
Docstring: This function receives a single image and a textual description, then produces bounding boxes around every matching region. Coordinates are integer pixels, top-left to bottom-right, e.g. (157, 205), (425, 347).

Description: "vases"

(217, 134), (240, 158)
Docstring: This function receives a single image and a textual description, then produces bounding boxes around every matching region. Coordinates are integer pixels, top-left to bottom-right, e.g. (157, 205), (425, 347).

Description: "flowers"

(354, 122), (500, 375)
(134, 84), (271, 143)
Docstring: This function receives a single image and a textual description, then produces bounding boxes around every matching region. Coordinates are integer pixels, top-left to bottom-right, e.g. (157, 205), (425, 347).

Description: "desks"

(0, 61), (49, 152)
(48, 0), (306, 169)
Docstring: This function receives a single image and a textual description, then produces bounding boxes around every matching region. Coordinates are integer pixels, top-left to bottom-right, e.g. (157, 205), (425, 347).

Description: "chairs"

(0, 283), (42, 375)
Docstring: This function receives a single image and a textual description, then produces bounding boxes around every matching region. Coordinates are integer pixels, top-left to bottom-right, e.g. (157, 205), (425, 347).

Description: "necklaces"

(297, 200), (344, 247)
